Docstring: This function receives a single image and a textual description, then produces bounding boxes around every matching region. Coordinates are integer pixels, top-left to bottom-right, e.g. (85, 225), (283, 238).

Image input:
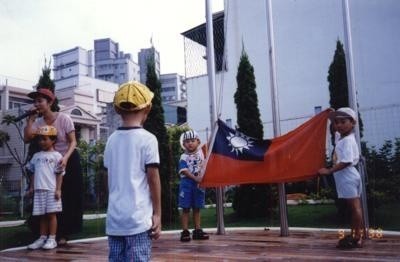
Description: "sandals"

(192, 229), (209, 239)
(181, 229), (190, 241)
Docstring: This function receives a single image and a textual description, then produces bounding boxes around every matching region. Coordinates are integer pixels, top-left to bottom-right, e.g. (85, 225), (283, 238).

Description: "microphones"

(14, 109), (40, 122)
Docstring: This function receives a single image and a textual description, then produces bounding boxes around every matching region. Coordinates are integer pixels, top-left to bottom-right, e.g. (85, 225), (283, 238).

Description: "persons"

(317, 106), (365, 248)
(178, 130), (216, 241)
(102, 82), (162, 261)
(26, 126), (66, 249)
(23, 88), (84, 246)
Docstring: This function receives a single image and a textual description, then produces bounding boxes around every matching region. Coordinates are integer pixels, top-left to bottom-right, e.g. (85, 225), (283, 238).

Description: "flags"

(197, 107), (335, 188)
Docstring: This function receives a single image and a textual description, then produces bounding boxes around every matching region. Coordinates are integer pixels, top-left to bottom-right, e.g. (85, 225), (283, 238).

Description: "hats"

(32, 126), (57, 136)
(183, 130), (198, 141)
(27, 88), (55, 100)
(327, 107), (356, 121)
(114, 81), (154, 111)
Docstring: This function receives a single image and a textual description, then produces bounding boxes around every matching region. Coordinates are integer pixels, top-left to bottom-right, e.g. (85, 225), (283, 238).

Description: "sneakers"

(42, 238), (57, 249)
(27, 238), (47, 249)
